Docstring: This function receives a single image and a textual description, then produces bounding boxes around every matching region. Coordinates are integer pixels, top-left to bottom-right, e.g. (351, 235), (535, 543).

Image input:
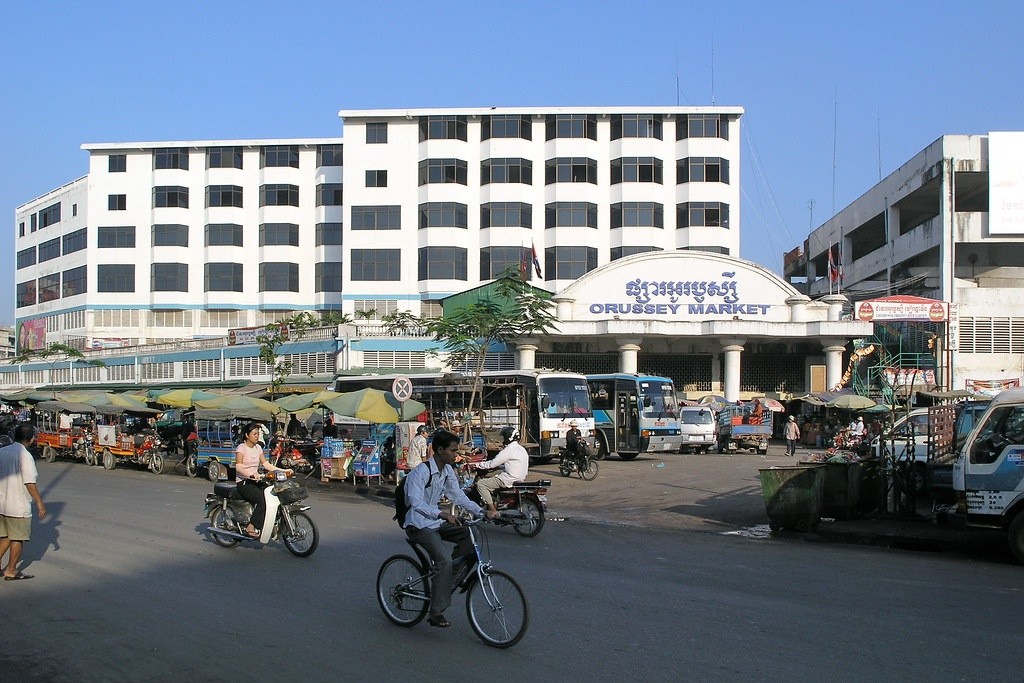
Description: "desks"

(321, 457), (348, 482)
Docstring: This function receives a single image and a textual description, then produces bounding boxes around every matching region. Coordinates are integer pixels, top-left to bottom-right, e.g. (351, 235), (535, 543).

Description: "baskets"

(278, 485), (309, 505)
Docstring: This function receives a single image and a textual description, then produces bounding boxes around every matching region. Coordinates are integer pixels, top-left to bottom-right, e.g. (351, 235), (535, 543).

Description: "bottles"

(322, 436), (344, 458)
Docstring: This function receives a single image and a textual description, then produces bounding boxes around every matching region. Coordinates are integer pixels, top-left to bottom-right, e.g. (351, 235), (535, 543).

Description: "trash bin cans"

(800, 461), (859, 521)
(759, 464), (824, 533)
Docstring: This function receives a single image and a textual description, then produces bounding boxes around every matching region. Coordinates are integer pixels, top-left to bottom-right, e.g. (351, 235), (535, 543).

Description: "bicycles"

(375, 515), (530, 650)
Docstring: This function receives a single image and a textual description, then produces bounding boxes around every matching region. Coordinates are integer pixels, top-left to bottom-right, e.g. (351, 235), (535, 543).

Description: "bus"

(583, 372), (681, 461)
(333, 366), (596, 465)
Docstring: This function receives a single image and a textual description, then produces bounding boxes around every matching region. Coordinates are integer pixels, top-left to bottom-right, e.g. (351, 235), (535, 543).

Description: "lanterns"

(916, 390), (994, 408)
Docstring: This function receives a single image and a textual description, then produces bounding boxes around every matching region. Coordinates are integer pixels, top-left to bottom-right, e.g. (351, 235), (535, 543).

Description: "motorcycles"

(450, 467), (552, 537)
(557, 438), (600, 481)
(33, 401), (275, 483)
(204, 470), (320, 558)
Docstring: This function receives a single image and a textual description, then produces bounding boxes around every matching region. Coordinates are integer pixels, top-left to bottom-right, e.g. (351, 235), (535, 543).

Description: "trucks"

(869, 406), (952, 493)
(952, 386), (1024, 567)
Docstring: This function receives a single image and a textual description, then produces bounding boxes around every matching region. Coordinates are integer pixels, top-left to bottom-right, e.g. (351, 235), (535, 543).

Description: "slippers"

(426, 614), (450, 628)
(244, 529), (256, 537)
(5, 571), (33, 581)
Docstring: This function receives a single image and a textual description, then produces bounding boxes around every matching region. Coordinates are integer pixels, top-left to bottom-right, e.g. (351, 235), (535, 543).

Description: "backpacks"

(394, 460), (448, 529)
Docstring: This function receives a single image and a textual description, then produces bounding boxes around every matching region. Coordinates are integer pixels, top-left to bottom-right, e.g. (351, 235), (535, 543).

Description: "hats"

(857, 417), (863, 420)
(569, 420), (580, 426)
(788, 415), (794, 421)
(451, 420), (462, 428)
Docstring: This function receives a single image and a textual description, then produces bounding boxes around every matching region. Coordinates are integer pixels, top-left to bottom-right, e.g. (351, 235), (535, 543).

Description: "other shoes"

(481, 512), (500, 521)
(785, 453), (789, 456)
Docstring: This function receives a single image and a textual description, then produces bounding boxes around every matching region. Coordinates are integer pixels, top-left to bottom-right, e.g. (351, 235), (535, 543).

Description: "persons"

(566, 420), (592, 471)
(557, 399), (589, 414)
(181, 411), (355, 465)
(0, 423), (47, 580)
(782, 415), (800, 457)
(471, 426), (529, 519)
(403, 431), (497, 627)
(737, 399), (762, 417)
(800, 411), (891, 451)
(0, 406), (167, 435)
(17, 324), (26, 356)
(381, 411), (473, 483)
(235, 423), (294, 538)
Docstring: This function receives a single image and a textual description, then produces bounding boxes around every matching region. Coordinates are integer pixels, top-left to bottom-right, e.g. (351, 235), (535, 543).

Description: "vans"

(715, 404), (774, 455)
(680, 406), (717, 454)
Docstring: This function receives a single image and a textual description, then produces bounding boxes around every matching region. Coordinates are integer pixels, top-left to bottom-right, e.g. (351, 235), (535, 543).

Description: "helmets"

(418, 426), (429, 432)
(499, 426), (520, 445)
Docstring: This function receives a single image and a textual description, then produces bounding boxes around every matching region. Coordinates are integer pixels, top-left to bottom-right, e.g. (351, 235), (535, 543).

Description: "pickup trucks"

(923, 403), (988, 507)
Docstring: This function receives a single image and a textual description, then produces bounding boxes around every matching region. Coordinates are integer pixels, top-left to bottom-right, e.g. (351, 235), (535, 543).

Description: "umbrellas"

(825, 387), (903, 426)
(697, 395), (734, 412)
(273, 388), (425, 424)
(748, 397), (785, 412)
(0, 388), (280, 416)
